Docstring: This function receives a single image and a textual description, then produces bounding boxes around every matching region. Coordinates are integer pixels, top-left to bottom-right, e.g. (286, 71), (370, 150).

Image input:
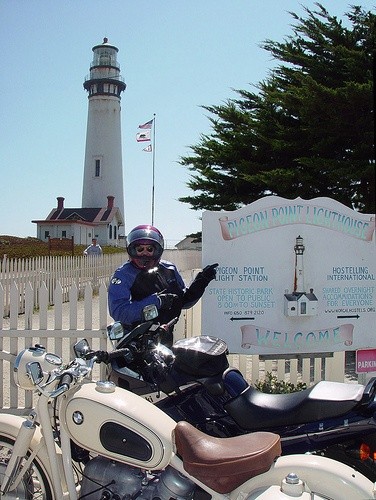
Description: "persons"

(108, 225), (219, 349)
(83, 239), (103, 257)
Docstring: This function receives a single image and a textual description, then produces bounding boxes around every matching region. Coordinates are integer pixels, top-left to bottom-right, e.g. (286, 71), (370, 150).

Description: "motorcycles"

(0, 338), (376, 500)
(73, 305), (376, 484)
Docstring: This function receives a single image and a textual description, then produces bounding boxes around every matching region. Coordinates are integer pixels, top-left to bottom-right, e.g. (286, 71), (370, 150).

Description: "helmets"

(127, 225), (164, 269)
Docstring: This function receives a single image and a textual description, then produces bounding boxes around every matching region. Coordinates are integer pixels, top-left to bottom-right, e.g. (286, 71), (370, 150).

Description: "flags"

(141, 144), (152, 152)
(139, 119), (153, 130)
(136, 130), (151, 142)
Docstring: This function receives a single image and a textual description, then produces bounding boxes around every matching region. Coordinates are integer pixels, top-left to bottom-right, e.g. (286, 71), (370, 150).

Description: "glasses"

(135, 247), (153, 252)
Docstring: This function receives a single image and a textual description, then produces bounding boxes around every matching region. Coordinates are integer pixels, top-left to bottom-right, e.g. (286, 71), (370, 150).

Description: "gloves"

(157, 289), (179, 310)
(192, 263), (219, 289)
